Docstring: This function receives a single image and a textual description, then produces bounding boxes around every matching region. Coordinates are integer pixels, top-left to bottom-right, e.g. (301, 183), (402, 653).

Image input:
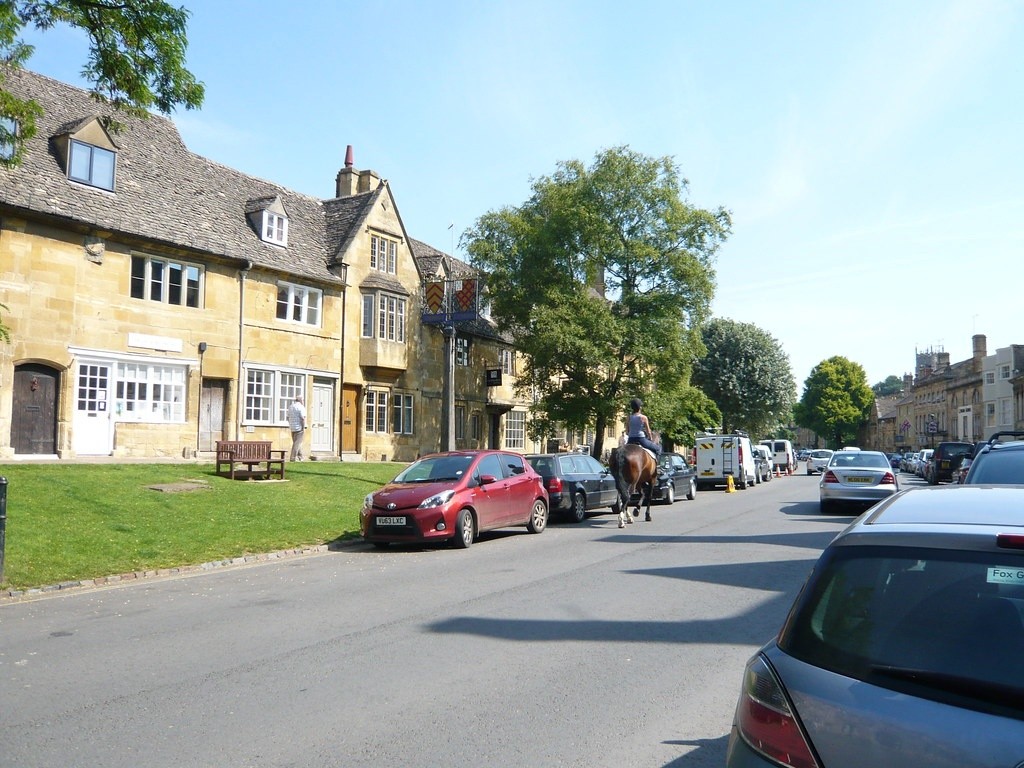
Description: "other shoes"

(290, 458), (296, 461)
(299, 458), (305, 461)
(656, 465), (664, 475)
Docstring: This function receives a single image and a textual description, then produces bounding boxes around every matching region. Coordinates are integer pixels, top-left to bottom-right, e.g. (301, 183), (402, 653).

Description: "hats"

(297, 395), (302, 398)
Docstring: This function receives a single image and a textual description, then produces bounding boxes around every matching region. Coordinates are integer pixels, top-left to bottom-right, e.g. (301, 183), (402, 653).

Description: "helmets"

(631, 398), (643, 409)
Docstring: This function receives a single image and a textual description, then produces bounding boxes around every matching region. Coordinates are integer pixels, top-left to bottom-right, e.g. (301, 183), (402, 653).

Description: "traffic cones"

(789, 463), (793, 475)
(784, 467), (788, 476)
(775, 463), (781, 478)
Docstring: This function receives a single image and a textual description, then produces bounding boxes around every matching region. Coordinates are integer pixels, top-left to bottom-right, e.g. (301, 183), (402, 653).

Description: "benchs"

(836, 458), (883, 467)
(852, 570), (1024, 690)
(215, 440), (287, 482)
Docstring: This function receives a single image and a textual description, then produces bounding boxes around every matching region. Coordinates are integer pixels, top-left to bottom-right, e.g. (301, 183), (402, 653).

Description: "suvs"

(964, 429), (1024, 486)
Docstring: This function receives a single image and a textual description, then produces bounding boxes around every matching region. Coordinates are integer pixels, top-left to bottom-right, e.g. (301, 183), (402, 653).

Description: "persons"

(625, 398), (661, 457)
(286, 396), (307, 461)
(618, 431), (629, 447)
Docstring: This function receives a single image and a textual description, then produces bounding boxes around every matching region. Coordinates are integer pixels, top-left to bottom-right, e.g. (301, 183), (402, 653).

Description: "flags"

(900, 419), (911, 432)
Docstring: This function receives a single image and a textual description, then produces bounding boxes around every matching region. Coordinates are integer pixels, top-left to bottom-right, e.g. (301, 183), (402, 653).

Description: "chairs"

(921, 561), (1022, 646)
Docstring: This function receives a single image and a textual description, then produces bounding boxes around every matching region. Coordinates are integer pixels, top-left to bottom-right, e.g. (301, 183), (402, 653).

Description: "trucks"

(694, 427), (760, 490)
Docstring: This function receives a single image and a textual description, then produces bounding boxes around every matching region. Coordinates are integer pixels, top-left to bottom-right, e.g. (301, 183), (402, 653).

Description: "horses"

(610, 430), (663, 530)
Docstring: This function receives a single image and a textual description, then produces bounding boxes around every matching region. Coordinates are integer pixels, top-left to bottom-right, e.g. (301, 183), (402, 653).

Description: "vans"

(752, 445), (774, 470)
(759, 439), (793, 470)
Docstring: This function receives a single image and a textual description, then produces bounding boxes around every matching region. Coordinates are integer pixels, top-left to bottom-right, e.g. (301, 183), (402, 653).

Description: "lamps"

(242, 260), (253, 273)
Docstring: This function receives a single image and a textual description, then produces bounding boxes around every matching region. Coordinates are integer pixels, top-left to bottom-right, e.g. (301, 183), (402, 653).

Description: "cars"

(359, 448), (549, 549)
(792, 446), (861, 471)
(752, 448), (772, 484)
(724, 483), (1023, 768)
(806, 449), (837, 476)
(817, 450), (900, 514)
(630, 452), (698, 505)
(521, 451), (625, 523)
(886, 440), (991, 485)
